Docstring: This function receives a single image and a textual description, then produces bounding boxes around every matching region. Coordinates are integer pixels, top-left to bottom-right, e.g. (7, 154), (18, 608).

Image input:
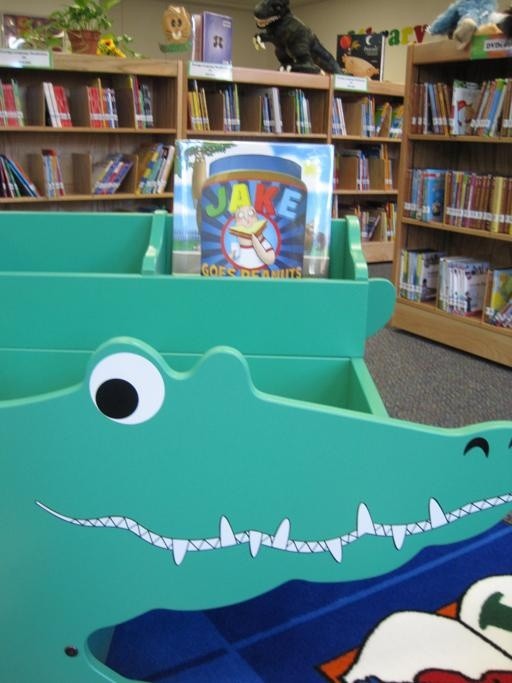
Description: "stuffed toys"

(252, 1), (354, 78)
(423, 0), (512, 51)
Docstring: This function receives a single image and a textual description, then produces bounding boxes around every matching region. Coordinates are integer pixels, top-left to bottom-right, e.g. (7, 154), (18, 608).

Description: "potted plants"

(51, 0), (120, 55)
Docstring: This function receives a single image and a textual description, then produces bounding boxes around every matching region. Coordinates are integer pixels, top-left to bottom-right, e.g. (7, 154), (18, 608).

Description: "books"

(331, 98), (404, 242)
(188, 79), (240, 132)
(190, 8), (234, 65)
(396, 72), (512, 331)
(261, 87), (311, 134)
(0, 80), (72, 128)
(93, 143), (174, 194)
(1, 149), (65, 197)
(85, 74), (154, 129)
(170, 137), (335, 282)
